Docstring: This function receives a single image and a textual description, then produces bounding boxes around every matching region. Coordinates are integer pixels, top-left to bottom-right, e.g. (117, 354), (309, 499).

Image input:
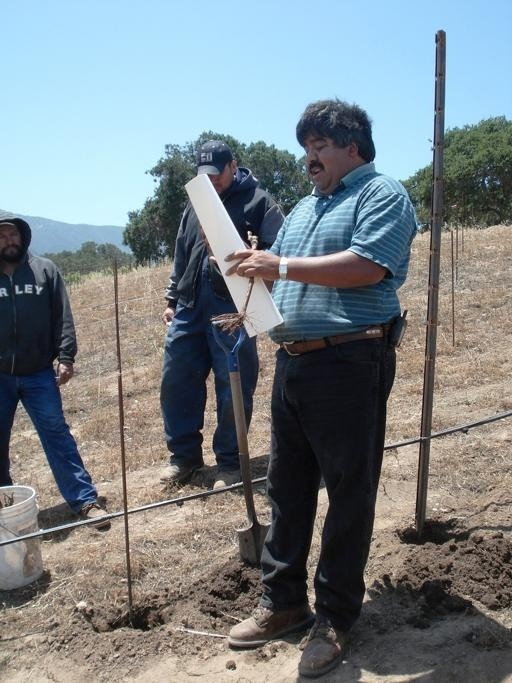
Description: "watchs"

(279, 256), (288, 281)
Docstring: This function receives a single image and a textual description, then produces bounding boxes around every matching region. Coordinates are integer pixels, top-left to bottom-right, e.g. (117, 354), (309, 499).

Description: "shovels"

(211, 317), (272, 565)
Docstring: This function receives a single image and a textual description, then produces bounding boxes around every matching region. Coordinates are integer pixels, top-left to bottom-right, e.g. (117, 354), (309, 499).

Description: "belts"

(279, 325), (384, 356)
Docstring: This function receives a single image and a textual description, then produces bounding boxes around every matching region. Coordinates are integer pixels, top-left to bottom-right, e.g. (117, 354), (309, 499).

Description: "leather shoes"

(80, 502), (110, 529)
(228, 603), (315, 649)
(159, 456), (204, 484)
(299, 614), (351, 677)
(212, 468), (241, 490)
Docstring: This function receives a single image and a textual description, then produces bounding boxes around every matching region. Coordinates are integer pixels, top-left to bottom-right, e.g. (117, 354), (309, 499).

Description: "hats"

(0, 222), (16, 226)
(196, 140), (234, 175)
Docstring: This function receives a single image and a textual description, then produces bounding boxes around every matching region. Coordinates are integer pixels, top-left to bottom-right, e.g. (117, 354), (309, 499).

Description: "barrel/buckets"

(0, 486), (41, 589)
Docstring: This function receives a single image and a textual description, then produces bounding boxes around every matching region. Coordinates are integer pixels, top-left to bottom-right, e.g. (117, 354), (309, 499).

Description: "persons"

(215, 98), (421, 678)
(158, 139), (286, 490)
(0, 209), (111, 529)
